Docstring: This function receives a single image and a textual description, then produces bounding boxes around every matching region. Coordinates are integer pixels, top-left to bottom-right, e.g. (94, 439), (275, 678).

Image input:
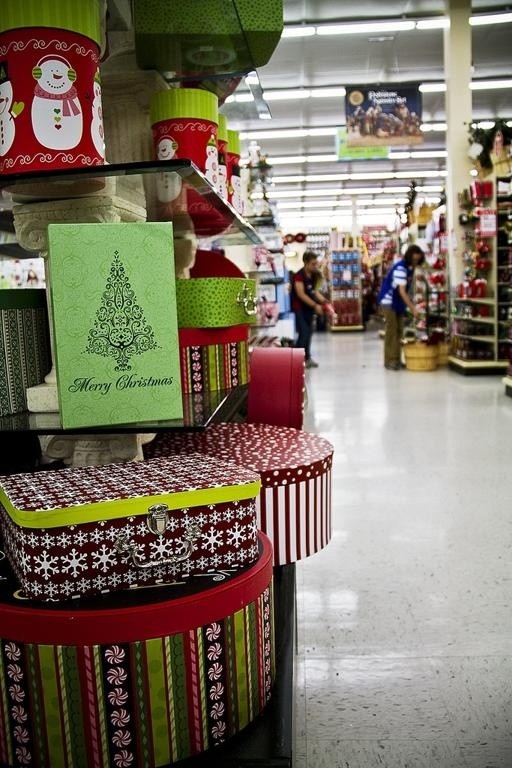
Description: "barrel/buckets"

(437, 340), (450, 365)
(403, 343), (439, 370)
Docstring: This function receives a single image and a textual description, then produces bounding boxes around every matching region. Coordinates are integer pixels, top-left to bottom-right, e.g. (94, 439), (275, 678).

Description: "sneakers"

(385, 362), (406, 371)
(305, 359), (319, 368)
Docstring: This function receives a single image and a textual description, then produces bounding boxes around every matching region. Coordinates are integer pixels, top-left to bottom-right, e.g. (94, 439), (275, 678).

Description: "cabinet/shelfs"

(325, 172), (512, 375)
(0, 0), (300, 768)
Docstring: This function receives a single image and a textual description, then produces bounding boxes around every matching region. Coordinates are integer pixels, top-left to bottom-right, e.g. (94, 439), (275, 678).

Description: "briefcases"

(176, 277), (259, 328)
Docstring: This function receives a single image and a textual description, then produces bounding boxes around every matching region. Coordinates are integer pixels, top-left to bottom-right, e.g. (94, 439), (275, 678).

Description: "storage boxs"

(1, 529), (274, 768)
(142, 422), (333, 566)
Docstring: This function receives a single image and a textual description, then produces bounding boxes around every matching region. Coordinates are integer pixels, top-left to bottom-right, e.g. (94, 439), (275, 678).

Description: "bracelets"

(312, 303), (317, 311)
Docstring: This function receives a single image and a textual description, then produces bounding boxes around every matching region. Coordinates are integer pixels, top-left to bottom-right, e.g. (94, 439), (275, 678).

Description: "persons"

(376, 244), (425, 371)
(289, 251), (334, 369)
